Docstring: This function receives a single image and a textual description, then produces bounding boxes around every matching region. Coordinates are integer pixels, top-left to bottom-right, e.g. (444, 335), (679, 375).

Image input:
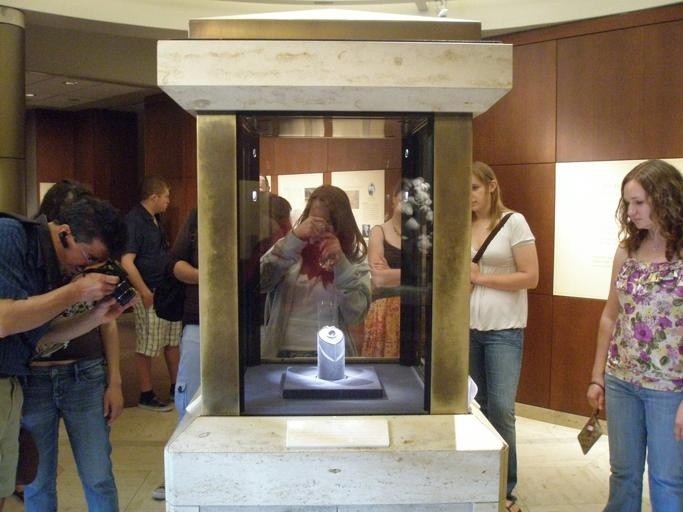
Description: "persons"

(257, 183), (371, 359)
(116, 170), (183, 412)
(464, 159), (540, 511)
(150, 204), (282, 502)
(585, 157), (682, 511)
(0, 191), (143, 511)
(20, 177), (124, 511)
(253, 171), (296, 237)
(358, 181), (410, 358)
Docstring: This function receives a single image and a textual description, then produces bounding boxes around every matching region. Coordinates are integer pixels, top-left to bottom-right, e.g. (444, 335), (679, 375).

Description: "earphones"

(58, 231), (67, 247)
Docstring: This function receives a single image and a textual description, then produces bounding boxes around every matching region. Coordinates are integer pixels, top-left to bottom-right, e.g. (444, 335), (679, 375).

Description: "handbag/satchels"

(578, 381), (605, 455)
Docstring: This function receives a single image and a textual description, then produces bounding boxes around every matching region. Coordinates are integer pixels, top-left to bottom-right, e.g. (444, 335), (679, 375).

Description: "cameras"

(92, 260), (134, 306)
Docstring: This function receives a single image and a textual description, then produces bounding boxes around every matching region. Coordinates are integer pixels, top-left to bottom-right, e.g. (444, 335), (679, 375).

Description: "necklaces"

(392, 219), (402, 240)
(644, 238), (668, 261)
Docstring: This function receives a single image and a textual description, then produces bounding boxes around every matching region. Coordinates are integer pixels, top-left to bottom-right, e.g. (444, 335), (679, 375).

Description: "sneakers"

(138, 395), (172, 412)
(153, 482), (166, 500)
